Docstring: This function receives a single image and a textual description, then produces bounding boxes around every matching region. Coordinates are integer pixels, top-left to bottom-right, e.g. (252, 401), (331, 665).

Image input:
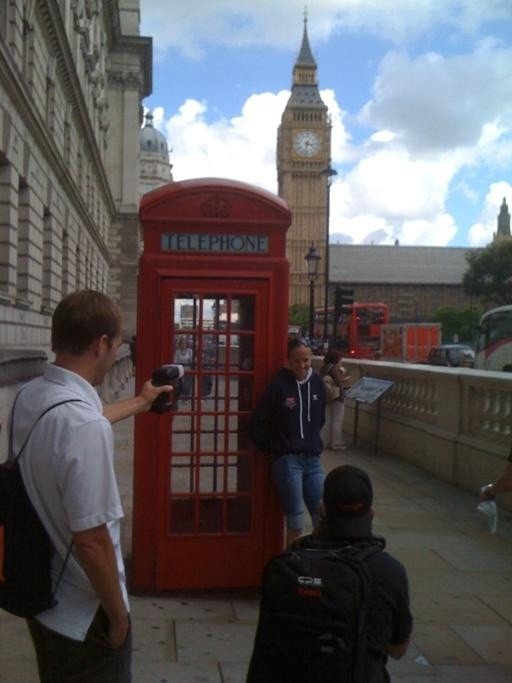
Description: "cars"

(426, 344), (475, 367)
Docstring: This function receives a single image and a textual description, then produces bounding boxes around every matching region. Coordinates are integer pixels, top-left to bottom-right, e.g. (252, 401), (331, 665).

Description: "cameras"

(148, 360), (185, 412)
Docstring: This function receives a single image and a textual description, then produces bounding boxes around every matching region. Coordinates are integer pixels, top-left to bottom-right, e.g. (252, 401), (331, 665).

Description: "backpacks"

(0, 386), (93, 619)
(322, 374), (340, 403)
(245, 541), (386, 683)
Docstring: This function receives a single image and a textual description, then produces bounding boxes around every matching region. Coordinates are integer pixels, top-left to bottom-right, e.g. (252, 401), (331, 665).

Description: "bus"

(311, 303), (390, 359)
(474, 304), (512, 373)
(215, 313), (241, 344)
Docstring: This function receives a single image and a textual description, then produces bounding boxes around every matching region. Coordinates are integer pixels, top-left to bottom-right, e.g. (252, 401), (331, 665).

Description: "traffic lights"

(335, 288), (354, 316)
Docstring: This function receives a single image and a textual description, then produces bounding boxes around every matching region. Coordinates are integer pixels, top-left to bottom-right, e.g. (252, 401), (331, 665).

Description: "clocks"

(293, 129), (320, 159)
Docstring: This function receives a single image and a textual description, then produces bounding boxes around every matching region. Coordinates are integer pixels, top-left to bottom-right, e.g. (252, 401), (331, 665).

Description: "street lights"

(318, 158), (338, 342)
(303, 241), (321, 341)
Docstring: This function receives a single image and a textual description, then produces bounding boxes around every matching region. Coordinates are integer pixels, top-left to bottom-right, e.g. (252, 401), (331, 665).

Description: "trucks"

(374, 321), (442, 363)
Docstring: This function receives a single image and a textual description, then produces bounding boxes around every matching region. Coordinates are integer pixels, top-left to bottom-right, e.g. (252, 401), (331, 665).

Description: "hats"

(322, 464), (373, 539)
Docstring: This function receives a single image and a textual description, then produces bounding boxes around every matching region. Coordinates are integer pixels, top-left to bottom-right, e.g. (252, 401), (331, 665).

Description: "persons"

(0, 288), (175, 682)
(132, 330), (217, 406)
(246, 462), (415, 682)
(318, 348), (352, 448)
(264, 333), (326, 547)
(474, 449), (511, 504)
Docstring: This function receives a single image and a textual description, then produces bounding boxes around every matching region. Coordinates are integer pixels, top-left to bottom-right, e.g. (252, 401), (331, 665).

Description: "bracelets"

(481, 483), (497, 498)
